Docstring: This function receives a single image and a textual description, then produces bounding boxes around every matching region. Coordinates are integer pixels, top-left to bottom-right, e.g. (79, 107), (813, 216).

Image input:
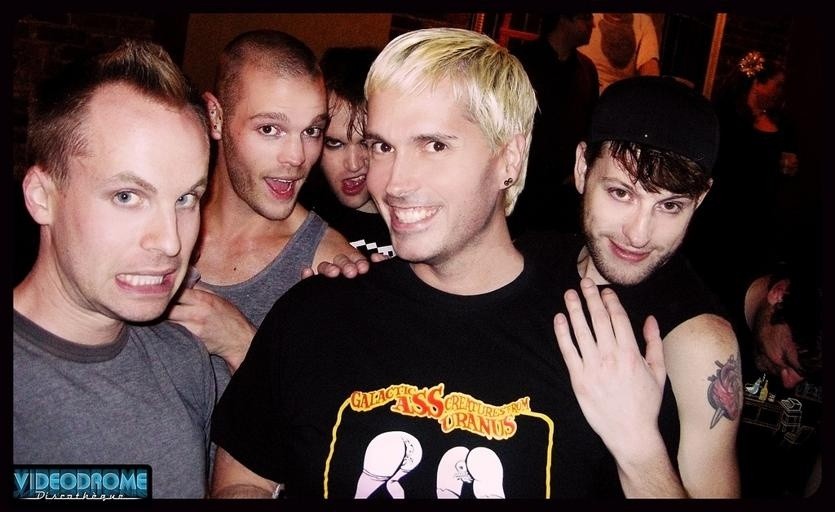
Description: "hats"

(591, 75), (722, 170)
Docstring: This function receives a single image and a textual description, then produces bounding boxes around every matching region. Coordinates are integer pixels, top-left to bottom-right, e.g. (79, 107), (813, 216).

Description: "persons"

(7, 1), (835, 501)
